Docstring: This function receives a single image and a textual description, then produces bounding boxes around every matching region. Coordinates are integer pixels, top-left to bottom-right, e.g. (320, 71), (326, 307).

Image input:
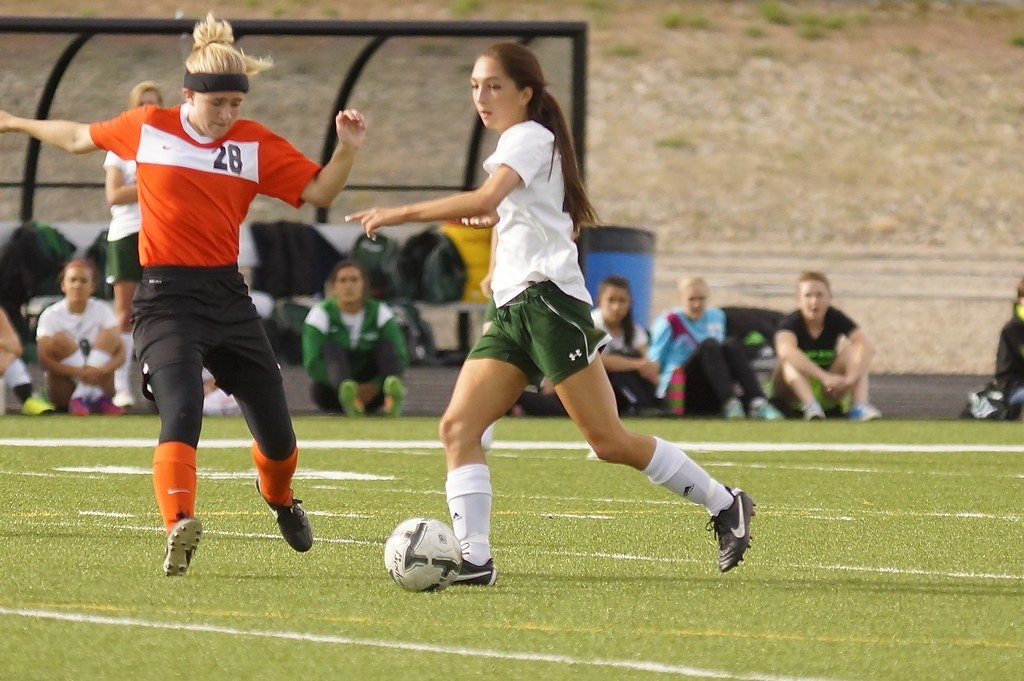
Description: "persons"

(484, 292), (566, 415)
(203, 221), (258, 397)
(303, 256), (407, 418)
(766, 271), (883, 420)
(995, 277), (1024, 419)
(0, 8), (367, 576)
(588, 274), (680, 418)
(346, 48), (756, 585)
(0, 306), (57, 417)
(36, 258), (126, 415)
(644, 277), (786, 420)
(102, 79), (164, 407)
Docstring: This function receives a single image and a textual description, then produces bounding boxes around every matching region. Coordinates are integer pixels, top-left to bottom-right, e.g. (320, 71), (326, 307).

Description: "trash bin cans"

(574, 226), (656, 334)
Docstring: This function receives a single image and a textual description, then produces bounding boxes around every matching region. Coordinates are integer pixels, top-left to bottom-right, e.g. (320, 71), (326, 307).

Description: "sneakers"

(445, 556), (498, 587)
(254, 475), (313, 552)
(19, 394), (55, 416)
(163, 512), (203, 578)
(705, 485), (757, 574)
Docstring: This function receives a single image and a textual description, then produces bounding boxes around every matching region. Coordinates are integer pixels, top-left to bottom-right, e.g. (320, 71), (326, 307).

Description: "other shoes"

(67, 397), (93, 418)
(748, 398), (782, 421)
(90, 396), (128, 416)
(722, 398), (744, 418)
(802, 402), (827, 420)
(338, 380), (366, 419)
(383, 376), (407, 419)
(846, 400), (880, 422)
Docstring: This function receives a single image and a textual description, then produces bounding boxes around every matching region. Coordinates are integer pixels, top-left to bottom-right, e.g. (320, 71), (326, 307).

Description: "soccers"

(384, 518), (464, 594)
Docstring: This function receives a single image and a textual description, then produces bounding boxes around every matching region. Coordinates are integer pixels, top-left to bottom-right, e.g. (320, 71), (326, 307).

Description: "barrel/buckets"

(574, 227), (654, 324)
(439, 210), (502, 304)
